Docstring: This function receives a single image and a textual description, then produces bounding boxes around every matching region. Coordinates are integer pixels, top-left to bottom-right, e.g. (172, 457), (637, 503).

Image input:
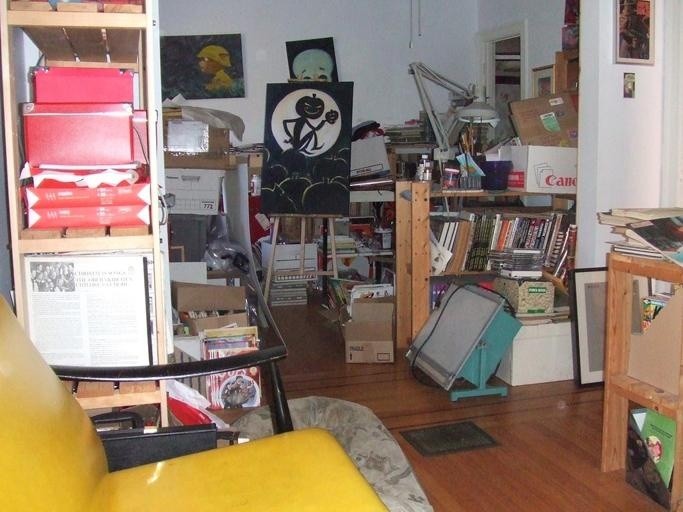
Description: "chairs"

(0, 291), (392, 512)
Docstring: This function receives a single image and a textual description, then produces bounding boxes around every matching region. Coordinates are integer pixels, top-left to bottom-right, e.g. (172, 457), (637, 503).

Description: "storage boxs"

(164, 168), (226, 216)
(497, 145), (577, 195)
(162, 123), (230, 168)
(493, 277), (555, 317)
(339, 298), (395, 365)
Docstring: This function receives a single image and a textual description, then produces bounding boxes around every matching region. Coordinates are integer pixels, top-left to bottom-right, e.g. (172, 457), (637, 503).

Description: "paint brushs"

(457, 116), (483, 155)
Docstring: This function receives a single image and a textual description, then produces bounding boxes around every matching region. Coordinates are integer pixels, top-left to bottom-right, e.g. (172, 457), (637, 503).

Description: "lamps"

(408, 61), (497, 163)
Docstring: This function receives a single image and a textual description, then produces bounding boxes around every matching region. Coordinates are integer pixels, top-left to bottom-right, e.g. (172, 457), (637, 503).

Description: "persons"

(197, 45), (233, 99)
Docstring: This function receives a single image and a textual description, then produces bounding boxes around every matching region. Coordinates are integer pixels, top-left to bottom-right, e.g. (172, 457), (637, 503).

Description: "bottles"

(250, 174), (257, 196)
(419, 154), (430, 167)
(423, 169), (432, 181)
(269, 217), (274, 244)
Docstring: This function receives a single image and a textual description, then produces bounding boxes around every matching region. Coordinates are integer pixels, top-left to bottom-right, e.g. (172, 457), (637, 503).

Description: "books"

(198, 323), (262, 410)
(327, 235), (358, 253)
(431, 210), (577, 275)
(597, 207), (683, 267)
(269, 281), (308, 306)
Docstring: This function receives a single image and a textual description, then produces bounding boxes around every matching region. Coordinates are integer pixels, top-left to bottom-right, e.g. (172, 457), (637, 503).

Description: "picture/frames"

(285, 36), (339, 81)
(611, 0), (657, 66)
(568, 267), (621, 388)
(161, 34), (246, 104)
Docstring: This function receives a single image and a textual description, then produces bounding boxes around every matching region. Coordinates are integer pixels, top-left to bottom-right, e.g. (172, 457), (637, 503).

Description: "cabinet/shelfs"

(0, 0), (170, 432)
(604, 251), (683, 511)
(320, 180), (580, 355)
